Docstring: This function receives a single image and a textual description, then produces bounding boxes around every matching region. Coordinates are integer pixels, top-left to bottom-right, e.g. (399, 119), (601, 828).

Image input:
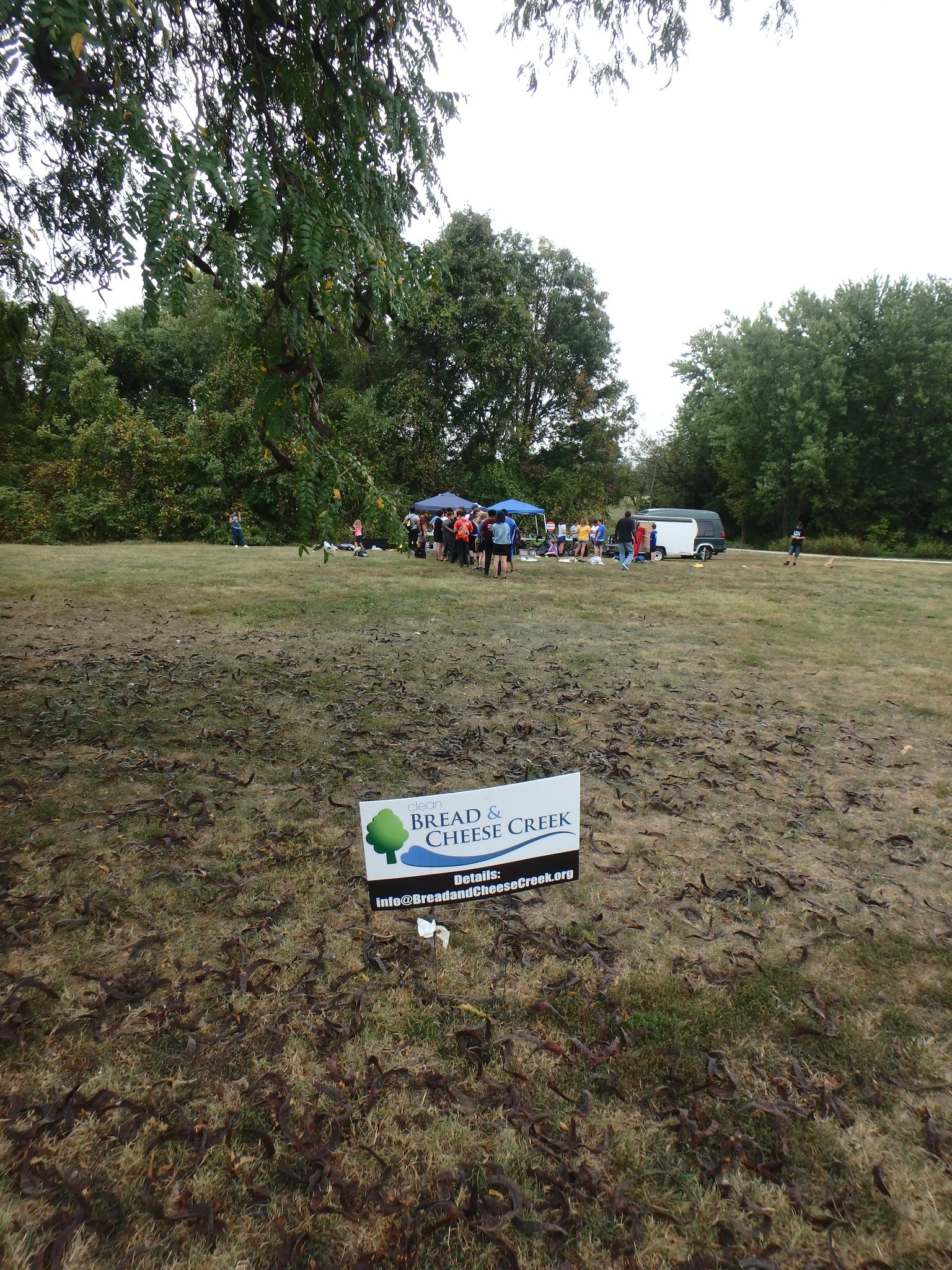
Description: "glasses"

(235, 511), (239, 513)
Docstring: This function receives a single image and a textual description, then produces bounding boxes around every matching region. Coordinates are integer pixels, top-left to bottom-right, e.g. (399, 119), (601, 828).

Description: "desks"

(418, 534), (573, 551)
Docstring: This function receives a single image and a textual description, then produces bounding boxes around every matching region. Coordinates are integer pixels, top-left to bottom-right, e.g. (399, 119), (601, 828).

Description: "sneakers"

(621, 563), (630, 571)
(235, 545), (238, 547)
(243, 545), (250, 548)
(474, 566), (515, 579)
(435, 559), (449, 562)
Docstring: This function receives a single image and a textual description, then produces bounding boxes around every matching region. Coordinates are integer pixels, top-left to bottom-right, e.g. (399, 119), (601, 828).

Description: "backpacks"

(406, 514), (417, 531)
(512, 524), (522, 547)
(458, 518), (469, 538)
(414, 547), (426, 559)
(354, 546), (368, 557)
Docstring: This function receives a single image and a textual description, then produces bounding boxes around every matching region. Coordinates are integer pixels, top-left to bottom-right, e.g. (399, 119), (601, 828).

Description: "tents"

(485, 499), (547, 535)
(410, 491), (486, 513)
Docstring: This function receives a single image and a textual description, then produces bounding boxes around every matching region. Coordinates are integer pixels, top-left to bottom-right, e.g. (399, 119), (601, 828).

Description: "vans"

(605, 508), (726, 560)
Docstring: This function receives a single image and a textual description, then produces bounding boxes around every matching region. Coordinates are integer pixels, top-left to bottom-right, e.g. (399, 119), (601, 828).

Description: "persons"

(783, 522), (806, 565)
(429, 503), (521, 579)
(544, 538), (558, 559)
(643, 524), (657, 563)
(403, 505), (422, 555)
(558, 518), (605, 558)
(350, 520), (366, 551)
(615, 510), (638, 571)
(417, 509), (429, 548)
(229, 508), (250, 548)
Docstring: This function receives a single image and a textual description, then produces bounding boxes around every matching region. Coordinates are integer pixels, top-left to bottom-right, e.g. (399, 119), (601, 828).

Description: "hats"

(797, 522), (802, 526)
(472, 503), (481, 508)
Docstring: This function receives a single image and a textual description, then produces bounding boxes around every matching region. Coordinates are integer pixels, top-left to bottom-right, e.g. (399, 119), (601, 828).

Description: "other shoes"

(784, 562), (790, 565)
(793, 563), (796, 565)
